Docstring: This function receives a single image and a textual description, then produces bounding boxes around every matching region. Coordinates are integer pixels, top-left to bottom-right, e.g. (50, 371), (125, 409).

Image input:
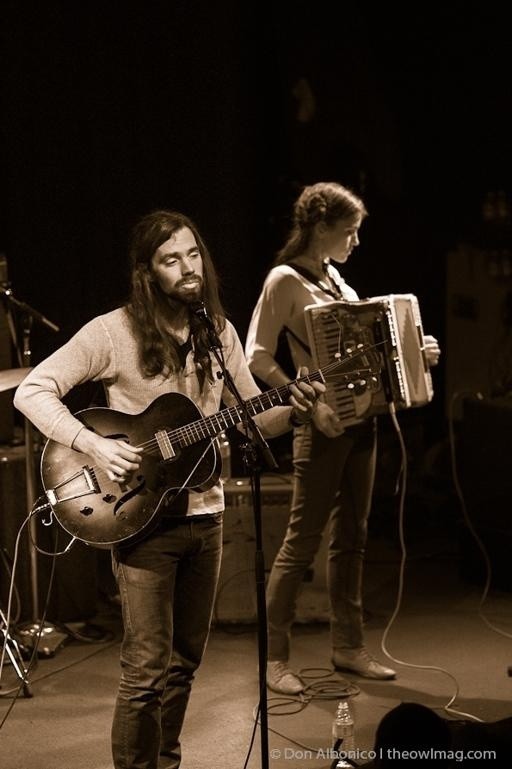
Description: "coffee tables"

(0, 366), (32, 392)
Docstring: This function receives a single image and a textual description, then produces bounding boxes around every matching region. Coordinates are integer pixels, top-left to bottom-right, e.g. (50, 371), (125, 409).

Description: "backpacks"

(0, 428), (54, 570)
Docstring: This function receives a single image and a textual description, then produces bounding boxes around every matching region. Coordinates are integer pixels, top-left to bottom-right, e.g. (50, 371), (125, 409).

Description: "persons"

(244, 180), (444, 699)
(12, 208), (333, 769)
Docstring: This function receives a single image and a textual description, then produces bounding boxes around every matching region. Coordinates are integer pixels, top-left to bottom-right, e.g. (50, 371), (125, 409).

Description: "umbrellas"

(330, 645), (396, 679)
(266, 661), (303, 695)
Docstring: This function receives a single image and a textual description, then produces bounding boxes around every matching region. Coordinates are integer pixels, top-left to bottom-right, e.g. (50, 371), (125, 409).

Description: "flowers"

(189, 298), (223, 349)
(0, 282), (59, 332)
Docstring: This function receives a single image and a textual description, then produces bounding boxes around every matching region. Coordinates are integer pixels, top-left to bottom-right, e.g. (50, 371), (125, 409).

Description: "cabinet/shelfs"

(331, 701), (355, 768)
